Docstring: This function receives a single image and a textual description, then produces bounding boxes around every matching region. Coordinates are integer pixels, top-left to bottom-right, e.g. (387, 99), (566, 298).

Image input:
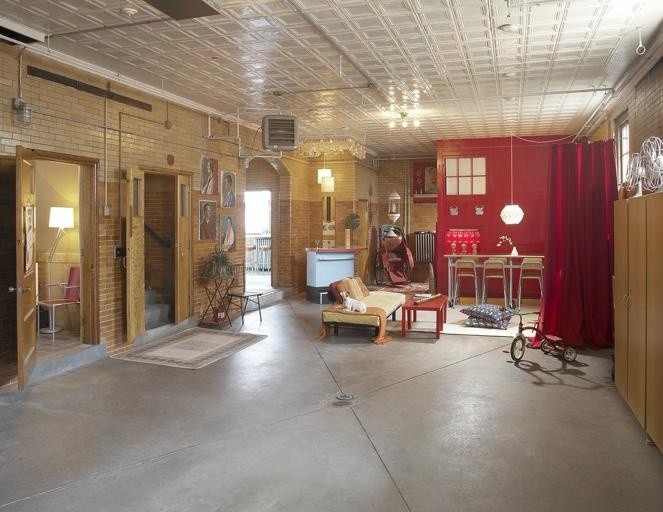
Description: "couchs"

(322, 277), (406, 339)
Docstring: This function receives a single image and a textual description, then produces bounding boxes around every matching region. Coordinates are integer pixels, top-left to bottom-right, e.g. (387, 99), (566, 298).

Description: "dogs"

(335, 291), (366, 313)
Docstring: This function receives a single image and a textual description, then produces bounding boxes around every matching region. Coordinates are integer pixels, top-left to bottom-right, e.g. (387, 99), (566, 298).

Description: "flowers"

(496, 235), (514, 248)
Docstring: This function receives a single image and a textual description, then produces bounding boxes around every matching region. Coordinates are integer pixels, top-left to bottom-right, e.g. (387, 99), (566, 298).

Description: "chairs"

(37, 266), (80, 344)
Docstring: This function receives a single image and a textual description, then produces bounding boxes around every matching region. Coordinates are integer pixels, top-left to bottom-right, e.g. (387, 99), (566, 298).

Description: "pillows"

(330, 277), (369, 304)
(464, 315), (512, 330)
(460, 303), (514, 323)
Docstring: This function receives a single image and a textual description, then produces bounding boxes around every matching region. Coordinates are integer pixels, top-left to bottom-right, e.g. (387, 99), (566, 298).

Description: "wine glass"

(313, 239), (321, 249)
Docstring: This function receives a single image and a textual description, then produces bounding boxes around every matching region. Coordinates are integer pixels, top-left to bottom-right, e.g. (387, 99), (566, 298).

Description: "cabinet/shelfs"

(612, 191), (663, 455)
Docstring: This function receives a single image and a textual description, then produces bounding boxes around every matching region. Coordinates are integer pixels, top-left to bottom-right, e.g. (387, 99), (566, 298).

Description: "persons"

(221, 216), (235, 252)
(200, 158), (217, 195)
(200, 203), (216, 239)
(221, 174), (235, 207)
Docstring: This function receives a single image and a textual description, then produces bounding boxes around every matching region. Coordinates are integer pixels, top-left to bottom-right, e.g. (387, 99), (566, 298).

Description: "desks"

(443, 254), (545, 307)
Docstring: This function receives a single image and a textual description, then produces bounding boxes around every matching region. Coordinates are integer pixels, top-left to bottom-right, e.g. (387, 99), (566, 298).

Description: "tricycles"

(501, 307), (578, 364)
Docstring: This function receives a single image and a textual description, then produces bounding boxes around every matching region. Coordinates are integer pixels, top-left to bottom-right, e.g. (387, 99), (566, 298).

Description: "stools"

(452, 256), (543, 311)
(246, 245), (256, 272)
(257, 246), (271, 273)
(225, 292), (263, 326)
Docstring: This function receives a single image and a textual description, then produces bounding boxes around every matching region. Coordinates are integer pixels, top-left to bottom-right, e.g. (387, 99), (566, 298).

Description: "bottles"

(450, 243), (477, 255)
(619, 187), (625, 200)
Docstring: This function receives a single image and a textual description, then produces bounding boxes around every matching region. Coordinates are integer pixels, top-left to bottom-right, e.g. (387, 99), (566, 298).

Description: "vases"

(511, 246), (518, 256)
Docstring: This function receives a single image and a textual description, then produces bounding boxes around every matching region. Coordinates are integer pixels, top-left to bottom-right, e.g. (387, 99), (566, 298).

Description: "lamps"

(318, 152), (331, 184)
(386, 191), (401, 224)
(321, 154), (334, 193)
(500, 127), (525, 225)
(39, 207), (74, 334)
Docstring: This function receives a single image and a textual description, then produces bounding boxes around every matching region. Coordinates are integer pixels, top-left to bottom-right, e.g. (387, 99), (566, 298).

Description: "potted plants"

(343, 213), (360, 250)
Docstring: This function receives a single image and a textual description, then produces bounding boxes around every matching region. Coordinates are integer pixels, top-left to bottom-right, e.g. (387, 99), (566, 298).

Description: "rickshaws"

(375, 223), (414, 287)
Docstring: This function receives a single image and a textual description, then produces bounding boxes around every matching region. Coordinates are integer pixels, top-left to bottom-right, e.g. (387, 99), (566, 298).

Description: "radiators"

(255, 236), (271, 269)
(415, 233), (435, 264)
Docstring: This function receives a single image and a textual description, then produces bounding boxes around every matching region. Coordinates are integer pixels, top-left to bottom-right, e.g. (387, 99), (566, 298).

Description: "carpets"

(109, 326), (268, 369)
(330, 281), (540, 338)
